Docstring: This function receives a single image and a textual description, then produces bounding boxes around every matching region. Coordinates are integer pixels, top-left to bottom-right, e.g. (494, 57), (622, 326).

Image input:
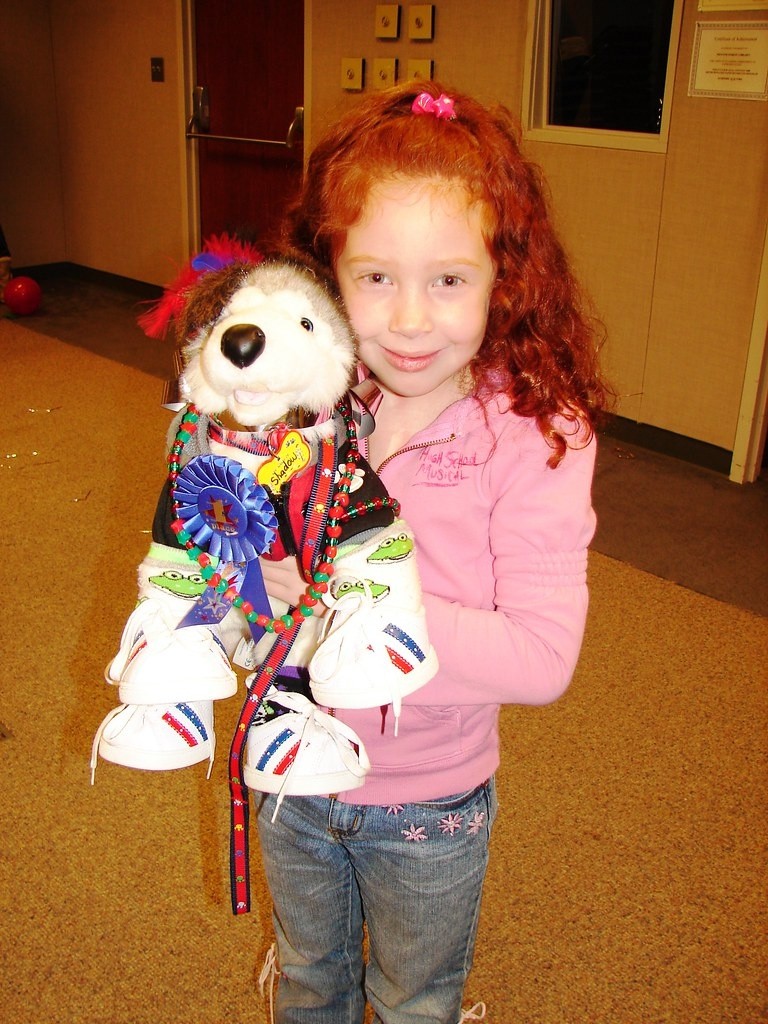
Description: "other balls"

(3, 276), (41, 315)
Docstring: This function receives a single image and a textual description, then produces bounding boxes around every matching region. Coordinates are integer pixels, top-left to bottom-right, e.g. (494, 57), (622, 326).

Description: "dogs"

(182, 254), (358, 432)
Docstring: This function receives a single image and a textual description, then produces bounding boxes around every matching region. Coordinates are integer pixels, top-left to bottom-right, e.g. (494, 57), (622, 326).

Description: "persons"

(248, 77), (598, 1024)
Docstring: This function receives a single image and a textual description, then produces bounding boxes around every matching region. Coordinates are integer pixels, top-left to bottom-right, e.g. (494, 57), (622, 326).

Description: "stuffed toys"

(98, 235), (440, 796)
